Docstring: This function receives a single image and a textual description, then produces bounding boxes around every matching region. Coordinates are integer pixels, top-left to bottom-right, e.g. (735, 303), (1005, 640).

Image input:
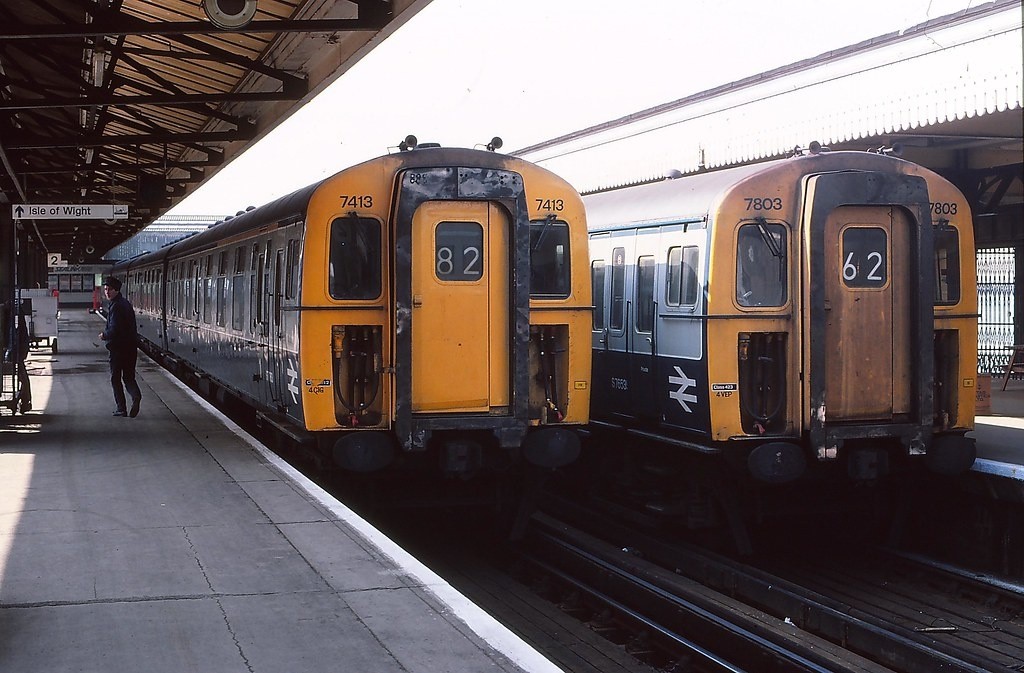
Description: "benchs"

(996, 363), (1024, 374)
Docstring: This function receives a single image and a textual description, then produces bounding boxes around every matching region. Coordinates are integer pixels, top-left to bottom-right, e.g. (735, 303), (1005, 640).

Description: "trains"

(103, 136), (593, 486)
(556, 141), (981, 524)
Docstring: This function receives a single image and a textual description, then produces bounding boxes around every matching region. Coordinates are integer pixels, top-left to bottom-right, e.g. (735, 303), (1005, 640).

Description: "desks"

(1000, 345), (1024, 392)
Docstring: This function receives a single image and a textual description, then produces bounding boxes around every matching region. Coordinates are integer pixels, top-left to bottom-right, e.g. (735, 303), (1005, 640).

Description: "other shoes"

(112, 409), (127, 417)
(130, 396), (140, 418)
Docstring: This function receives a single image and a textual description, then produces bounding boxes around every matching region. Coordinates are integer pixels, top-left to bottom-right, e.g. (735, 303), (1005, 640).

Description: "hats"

(102, 276), (122, 291)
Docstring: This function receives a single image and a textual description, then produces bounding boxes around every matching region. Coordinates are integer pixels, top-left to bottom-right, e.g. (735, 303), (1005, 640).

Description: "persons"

(98, 277), (142, 419)
